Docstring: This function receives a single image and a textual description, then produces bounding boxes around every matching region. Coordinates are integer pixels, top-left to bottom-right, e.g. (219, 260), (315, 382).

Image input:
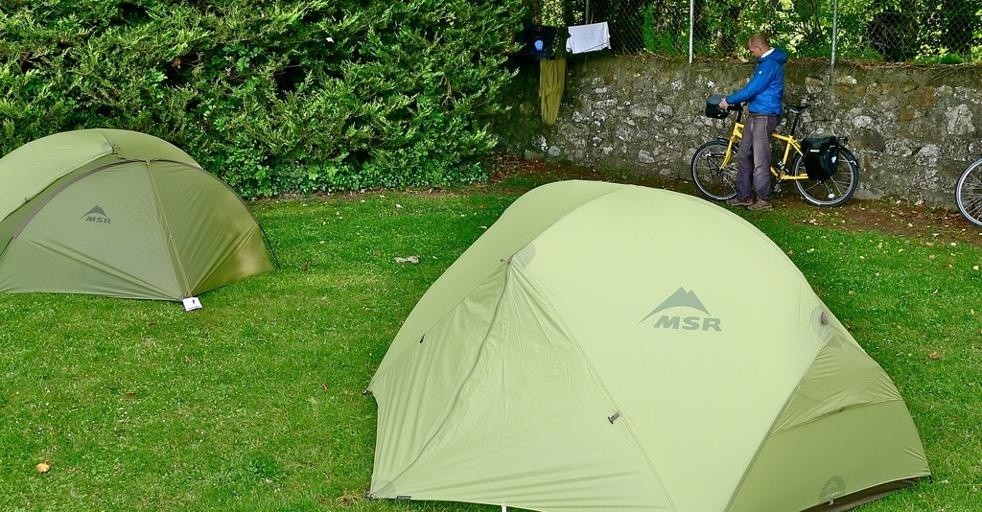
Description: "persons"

(716, 32), (790, 213)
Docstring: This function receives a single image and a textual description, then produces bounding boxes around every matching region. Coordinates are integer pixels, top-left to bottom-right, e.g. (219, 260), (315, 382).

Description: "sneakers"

(725, 197), (772, 211)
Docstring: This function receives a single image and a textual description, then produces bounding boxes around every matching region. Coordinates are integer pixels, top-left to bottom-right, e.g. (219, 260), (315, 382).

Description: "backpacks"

(800, 135), (838, 180)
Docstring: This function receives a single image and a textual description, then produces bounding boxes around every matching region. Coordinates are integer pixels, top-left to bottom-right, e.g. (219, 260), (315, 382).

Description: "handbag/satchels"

(705, 94), (728, 120)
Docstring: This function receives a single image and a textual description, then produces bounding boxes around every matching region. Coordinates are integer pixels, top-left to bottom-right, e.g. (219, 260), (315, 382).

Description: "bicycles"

(691, 94), (860, 208)
(954, 158), (982, 228)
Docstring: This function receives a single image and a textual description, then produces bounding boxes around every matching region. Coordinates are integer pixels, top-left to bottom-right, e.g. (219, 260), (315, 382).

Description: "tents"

(359, 177), (932, 512)
(0, 128), (282, 306)
